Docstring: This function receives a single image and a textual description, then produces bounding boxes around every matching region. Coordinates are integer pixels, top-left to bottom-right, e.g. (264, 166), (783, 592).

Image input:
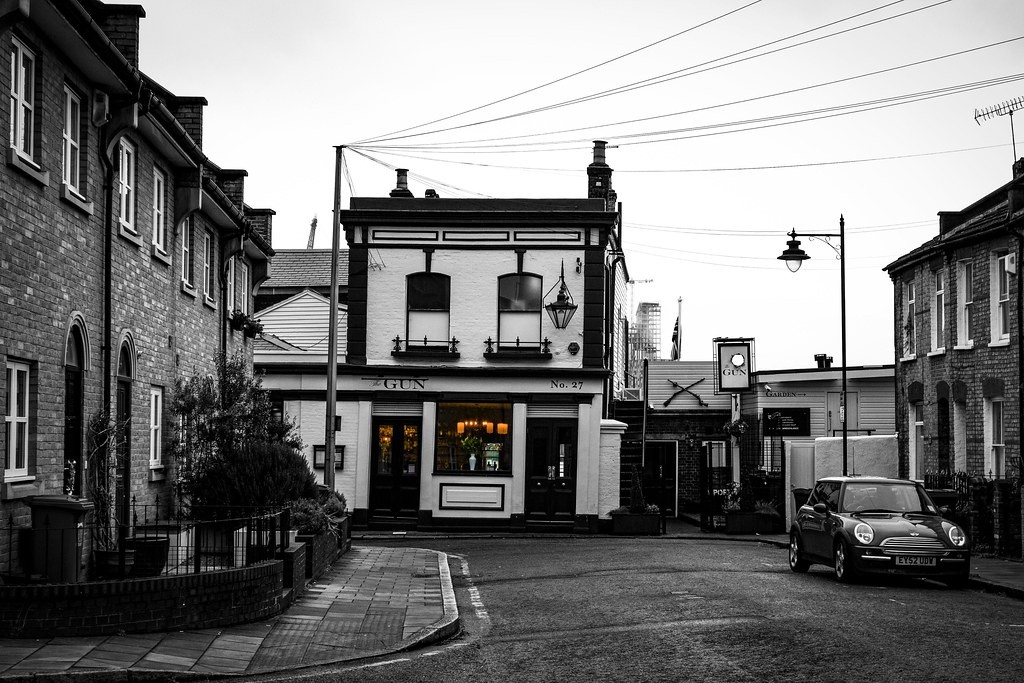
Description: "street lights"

(777, 213), (848, 476)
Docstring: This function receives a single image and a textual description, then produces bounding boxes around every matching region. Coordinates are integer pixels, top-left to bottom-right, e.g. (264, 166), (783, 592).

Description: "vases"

(246, 329), (256, 338)
(231, 318), (244, 331)
(610, 514), (660, 536)
(725, 512), (774, 535)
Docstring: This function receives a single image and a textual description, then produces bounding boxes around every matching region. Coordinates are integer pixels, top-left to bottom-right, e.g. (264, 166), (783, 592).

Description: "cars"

(788, 475), (972, 588)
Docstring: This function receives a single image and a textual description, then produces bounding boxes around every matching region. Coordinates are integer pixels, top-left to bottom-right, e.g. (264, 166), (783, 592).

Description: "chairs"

(877, 494), (904, 510)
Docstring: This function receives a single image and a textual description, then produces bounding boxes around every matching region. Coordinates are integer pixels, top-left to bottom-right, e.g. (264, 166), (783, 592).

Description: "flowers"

(246, 319), (264, 334)
(721, 467), (780, 517)
(606, 465), (660, 514)
(231, 309), (252, 325)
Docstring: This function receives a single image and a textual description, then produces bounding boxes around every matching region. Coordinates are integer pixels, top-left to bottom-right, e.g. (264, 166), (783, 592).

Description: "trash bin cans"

(21, 494), (96, 582)
(925, 488), (957, 523)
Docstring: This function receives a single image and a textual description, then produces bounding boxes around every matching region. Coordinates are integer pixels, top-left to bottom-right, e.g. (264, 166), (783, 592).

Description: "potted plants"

(460, 436), (482, 470)
(85, 408), (135, 581)
(125, 536), (171, 578)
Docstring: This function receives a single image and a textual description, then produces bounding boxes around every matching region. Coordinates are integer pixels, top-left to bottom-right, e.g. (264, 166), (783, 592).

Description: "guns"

(663, 377), (705, 406)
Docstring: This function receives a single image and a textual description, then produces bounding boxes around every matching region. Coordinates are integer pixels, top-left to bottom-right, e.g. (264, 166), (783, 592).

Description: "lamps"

(543, 258), (578, 329)
